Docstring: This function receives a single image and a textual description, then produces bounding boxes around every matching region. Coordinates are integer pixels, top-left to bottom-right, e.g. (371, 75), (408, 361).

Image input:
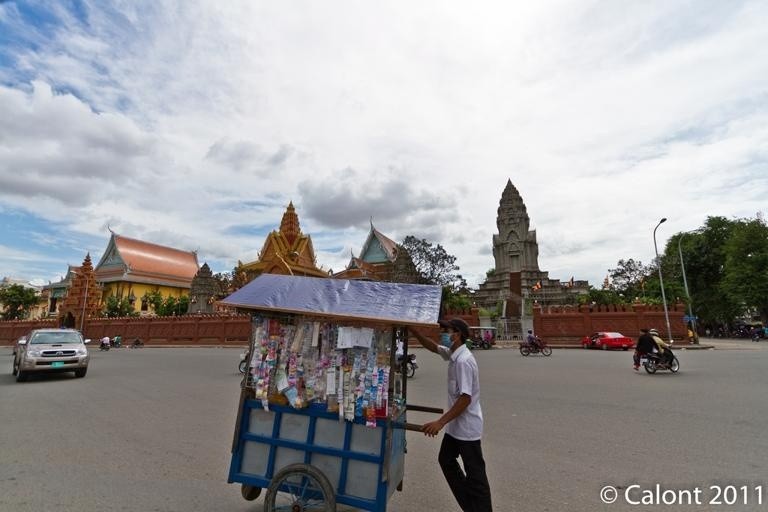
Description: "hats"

(440, 318), (470, 338)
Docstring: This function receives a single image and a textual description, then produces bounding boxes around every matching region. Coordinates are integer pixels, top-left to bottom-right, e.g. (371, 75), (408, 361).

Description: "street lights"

(653, 216), (676, 346)
(290, 252), (307, 277)
(677, 224), (714, 343)
(70, 270), (88, 337)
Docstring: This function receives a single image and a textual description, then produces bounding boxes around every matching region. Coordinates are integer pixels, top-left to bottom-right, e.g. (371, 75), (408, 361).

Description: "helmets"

(528, 329), (532, 334)
(650, 328), (659, 336)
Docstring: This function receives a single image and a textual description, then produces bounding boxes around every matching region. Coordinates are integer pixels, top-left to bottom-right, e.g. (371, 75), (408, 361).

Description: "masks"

(440, 332), (462, 349)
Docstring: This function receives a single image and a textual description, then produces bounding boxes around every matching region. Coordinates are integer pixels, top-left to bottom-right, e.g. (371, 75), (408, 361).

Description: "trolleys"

(226, 359), (445, 512)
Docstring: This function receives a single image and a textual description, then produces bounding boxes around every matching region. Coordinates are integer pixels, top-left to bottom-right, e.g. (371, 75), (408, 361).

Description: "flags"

(639, 277), (645, 289)
(3, 293), (214, 313)
(567, 276), (574, 288)
(600, 276), (609, 289)
(531, 280), (543, 292)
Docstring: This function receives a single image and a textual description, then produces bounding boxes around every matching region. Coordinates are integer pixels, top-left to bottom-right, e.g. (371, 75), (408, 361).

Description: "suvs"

(11, 327), (93, 382)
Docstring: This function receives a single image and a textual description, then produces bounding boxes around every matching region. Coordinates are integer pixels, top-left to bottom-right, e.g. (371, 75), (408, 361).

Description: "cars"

(581, 330), (634, 352)
(12, 335), (28, 355)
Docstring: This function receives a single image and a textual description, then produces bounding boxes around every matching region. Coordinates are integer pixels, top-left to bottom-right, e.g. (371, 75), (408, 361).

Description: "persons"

(99, 334), (141, 348)
(592, 333), (598, 346)
(649, 328), (673, 368)
(408, 318), (494, 512)
(632, 329), (659, 371)
(687, 322), (768, 342)
(395, 328), (540, 367)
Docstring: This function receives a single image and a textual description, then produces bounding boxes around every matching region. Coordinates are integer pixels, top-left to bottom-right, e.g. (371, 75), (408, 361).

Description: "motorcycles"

(749, 331), (760, 342)
(641, 337), (680, 375)
(99, 340), (111, 351)
(109, 339), (122, 349)
(393, 346), (420, 378)
(518, 338), (553, 357)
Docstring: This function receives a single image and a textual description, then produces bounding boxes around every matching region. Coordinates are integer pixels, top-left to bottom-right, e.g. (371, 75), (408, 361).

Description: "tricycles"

(465, 325), (499, 351)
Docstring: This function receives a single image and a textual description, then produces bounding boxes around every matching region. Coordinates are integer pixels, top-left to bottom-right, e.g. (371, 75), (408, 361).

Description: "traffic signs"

(683, 316), (699, 320)
